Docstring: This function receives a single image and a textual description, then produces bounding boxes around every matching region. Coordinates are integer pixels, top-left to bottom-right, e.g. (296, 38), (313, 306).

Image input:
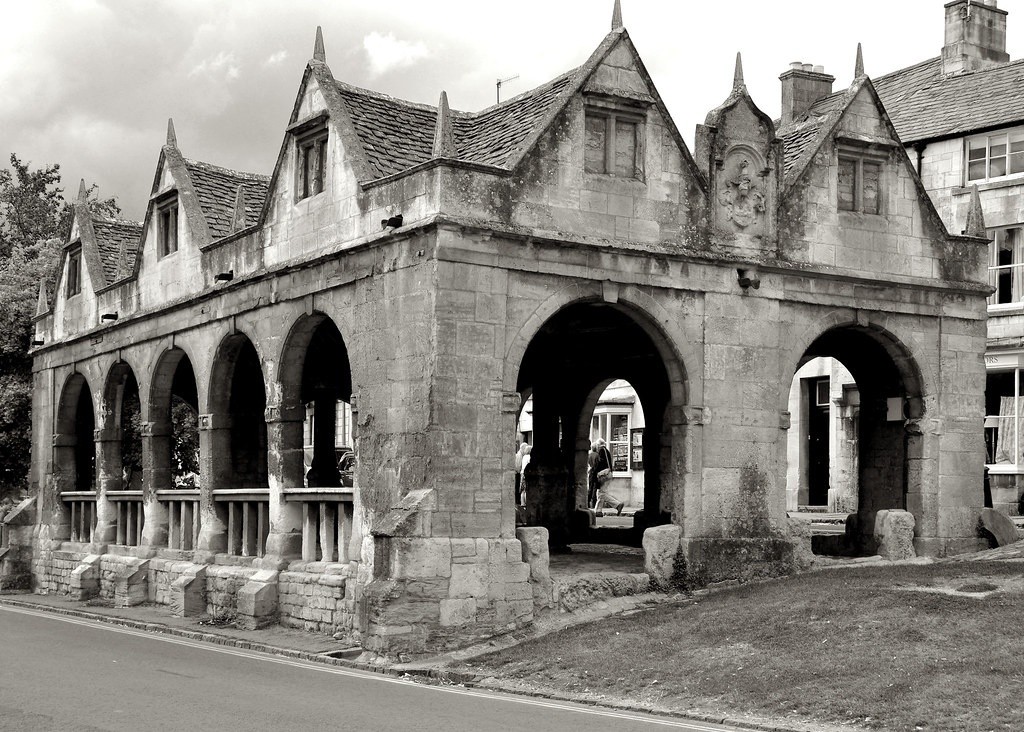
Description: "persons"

(515, 441), (528, 507)
(588, 438), (624, 518)
(587, 442), (601, 509)
(519, 445), (534, 510)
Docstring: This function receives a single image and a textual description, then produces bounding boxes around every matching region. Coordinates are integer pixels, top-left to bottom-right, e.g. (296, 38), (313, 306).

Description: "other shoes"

(616, 504), (624, 516)
(596, 512), (603, 518)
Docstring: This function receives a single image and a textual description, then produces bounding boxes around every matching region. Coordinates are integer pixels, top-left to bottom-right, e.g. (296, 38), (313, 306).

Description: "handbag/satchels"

(597, 468), (612, 482)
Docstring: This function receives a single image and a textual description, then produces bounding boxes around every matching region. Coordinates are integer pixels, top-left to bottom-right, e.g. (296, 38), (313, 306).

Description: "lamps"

(984, 415), (999, 463)
